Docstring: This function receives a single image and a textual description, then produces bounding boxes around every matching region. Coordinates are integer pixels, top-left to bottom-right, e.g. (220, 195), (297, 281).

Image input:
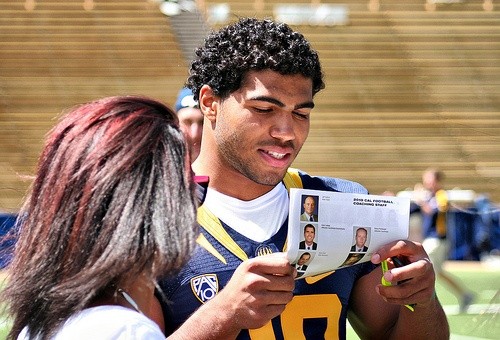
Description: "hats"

(176, 88), (200, 110)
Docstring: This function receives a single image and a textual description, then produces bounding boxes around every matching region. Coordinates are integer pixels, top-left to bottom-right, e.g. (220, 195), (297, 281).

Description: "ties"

(297, 267), (300, 270)
(358, 249), (362, 252)
(307, 247), (310, 250)
(310, 217), (313, 222)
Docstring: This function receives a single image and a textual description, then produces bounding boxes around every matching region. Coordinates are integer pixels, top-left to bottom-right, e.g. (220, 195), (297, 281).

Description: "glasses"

(381, 256), (415, 312)
(192, 176), (209, 207)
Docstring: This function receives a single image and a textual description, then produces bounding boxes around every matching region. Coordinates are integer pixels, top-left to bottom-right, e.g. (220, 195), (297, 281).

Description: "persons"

(409, 168), (479, 314)
(299, 223), (317, 250)
(0, 86), (203, 340)
(291, 252), (311, 271)
(300, 196), (318, 222)
(148, 18), (450, 340)
(342, 254), (360, 266)
(350, 226), (369, 252)
